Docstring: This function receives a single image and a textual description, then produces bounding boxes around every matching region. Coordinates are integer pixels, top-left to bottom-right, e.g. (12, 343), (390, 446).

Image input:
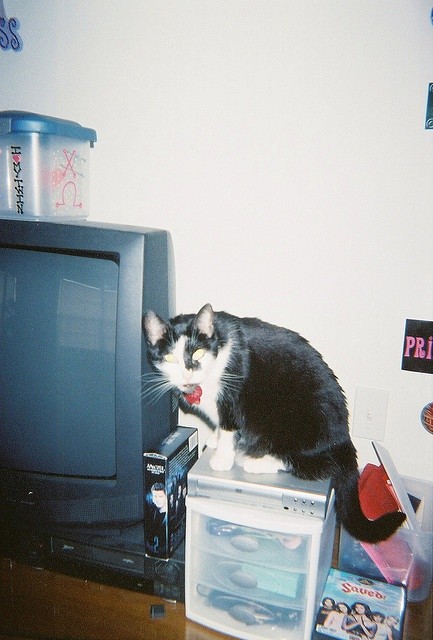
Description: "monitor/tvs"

(2, 220), (169, 539)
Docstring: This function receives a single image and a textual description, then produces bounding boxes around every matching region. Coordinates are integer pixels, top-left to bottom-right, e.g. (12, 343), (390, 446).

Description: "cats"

(142, 304), (406, 544)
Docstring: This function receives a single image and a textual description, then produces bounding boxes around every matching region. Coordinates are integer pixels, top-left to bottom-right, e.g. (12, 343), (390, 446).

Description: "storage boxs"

(0, 109), (97, 227)
(338, 476), (433, 605)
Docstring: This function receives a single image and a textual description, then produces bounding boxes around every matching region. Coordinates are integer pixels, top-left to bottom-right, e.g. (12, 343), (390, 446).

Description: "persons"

(323, 602), (352, 630)
(175, 483), (182, 516)
(371, 611), (393, 640)
(167, 492), (175, 522)
(385, 615), (402, 640)
(316, 597), (335, 625)
(341, 601), (377, 640)
(182, 484), (187, 513)
(145, 482), (168, 545)
